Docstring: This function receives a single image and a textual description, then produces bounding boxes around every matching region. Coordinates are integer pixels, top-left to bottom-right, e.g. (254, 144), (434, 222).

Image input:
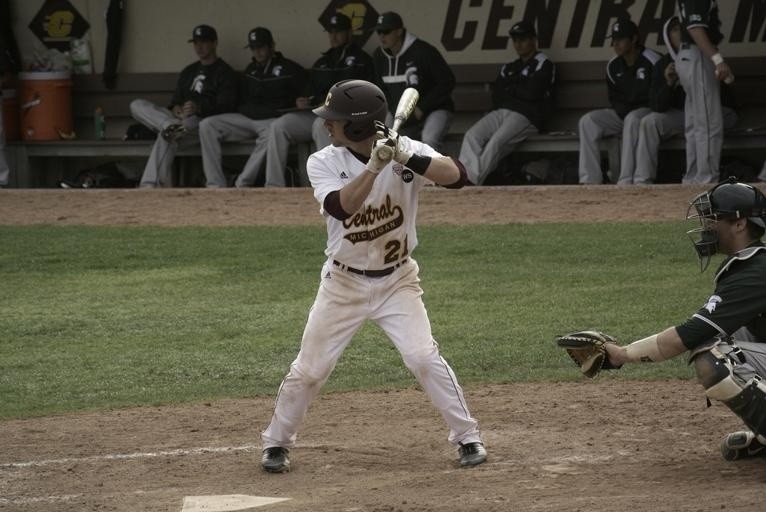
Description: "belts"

(332, 259), (406, 278)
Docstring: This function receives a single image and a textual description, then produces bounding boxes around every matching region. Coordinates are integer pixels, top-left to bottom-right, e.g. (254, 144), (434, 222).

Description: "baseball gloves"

(558, 331), (622, 377)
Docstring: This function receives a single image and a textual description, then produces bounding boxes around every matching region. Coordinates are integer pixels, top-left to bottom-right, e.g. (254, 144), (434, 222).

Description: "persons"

(260, 80), (486, 472)
(558, 176), (766, 460)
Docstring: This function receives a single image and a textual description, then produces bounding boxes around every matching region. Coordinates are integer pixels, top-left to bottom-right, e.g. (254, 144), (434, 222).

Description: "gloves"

(366, 139), (393, 176)
(374, 120), (414, 166)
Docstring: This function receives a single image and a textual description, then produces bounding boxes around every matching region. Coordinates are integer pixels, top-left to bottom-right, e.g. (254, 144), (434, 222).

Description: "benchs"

(0, 118), (766, 181)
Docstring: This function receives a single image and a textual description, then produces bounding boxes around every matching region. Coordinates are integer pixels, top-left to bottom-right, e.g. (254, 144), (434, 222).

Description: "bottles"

(94, 106), (105, 140)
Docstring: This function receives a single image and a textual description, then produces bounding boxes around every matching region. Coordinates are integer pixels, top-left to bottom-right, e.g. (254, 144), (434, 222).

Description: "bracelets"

(626, 334), (665, 369)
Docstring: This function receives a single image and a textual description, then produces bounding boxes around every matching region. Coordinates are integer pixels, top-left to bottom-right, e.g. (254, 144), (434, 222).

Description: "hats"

(322, 12), (351, 32)
(370, 12), (402, 31)
(187, 26), (217, 44)
(244, 27), (273, 48)
(605, 20), (638, 40)
(508, 22), (536, 39)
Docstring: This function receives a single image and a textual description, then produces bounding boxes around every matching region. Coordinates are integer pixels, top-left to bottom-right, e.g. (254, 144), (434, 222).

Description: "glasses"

(378, 30), (392, 34)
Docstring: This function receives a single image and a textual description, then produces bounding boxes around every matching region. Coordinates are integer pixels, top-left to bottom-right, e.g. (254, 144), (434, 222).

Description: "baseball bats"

(376, 86), (419, 162)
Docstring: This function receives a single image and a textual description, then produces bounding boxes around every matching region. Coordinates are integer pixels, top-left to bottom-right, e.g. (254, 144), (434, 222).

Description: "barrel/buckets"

(18, 69), (74, 143)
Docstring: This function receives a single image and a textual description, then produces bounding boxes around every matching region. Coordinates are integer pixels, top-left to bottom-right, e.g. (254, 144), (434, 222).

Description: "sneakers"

(457, 441), (487, 467)
(721, 431), (766, 461)
(160, 123), (199, 147)
(260, 448), (291, 473)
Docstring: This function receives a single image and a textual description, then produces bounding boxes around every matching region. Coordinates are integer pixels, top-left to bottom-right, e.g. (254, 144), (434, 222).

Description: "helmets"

(311, 79), (387, 143)
(682, 175), (766, 274)
